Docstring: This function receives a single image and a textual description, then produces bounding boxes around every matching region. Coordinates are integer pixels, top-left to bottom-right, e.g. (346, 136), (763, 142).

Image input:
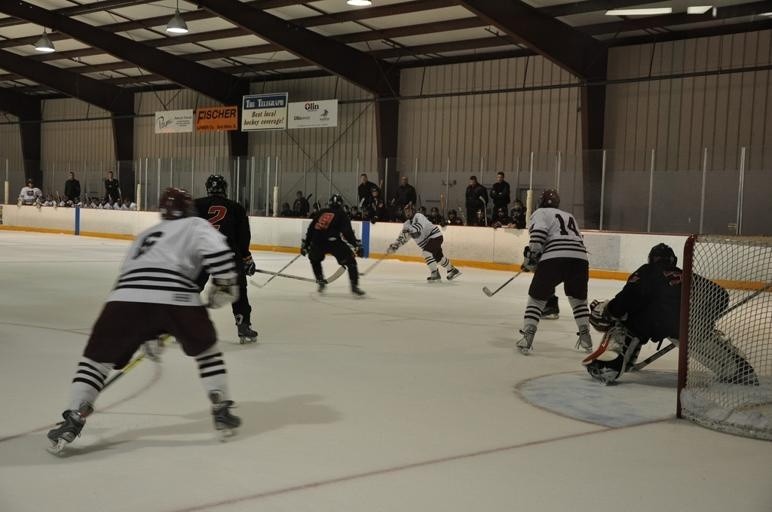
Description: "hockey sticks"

(479, 195), (488, 228)
(255, 264), (347, 285)
(358, 252), (388, 276)
(248, 253), (303, 289)
(482, 267), (526, 298)
(626, 282), (772, 374)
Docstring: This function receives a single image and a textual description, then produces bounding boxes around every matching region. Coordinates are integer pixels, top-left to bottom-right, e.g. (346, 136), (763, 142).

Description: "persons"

(502, 209), (523, 228)
(391, 201), (463, 282)
(368, 186), (384, 224)
(479, 207), (492, 226)
(342, 205), (351, 219)
(417, 206), (431, 223)
(310, 202), (321, 218)
(465, 175), (486, 225)
(357, 175), (381, 218)
(42, 192), (137, 210)
(18, 178), (41, 209)
(446, 208), (463, 224)
(64, 171), (81, 202)
(192, 174), (259, 344)
(300, 193), (366, 296)
(493, 206), (510, 226)
(279, 202), (295, 217)
(514, 198), (526, 210)
(516, 188), (595, 356)
(105, 170), (119, 203)
(392, 176), (417, 204)
(581, 242), (761, 387)
(292, 191), (310, 217)
(349, 206), (360, 219)
(45, 186), (242, 458)
(430, 207), (442, 224)
(539, 288), (560, 321)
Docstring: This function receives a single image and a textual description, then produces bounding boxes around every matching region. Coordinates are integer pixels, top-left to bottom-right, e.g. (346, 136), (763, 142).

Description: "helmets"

(431, 207), (437, 212)
(205, 175), (226, 195)
(496, 208), (504, 213)
(538, 190), (560, 208)
(159, 187), (190, 219)
(329, 194), (343, 208)
(403, 204), (415, 211)
(26, 178), (35, 185)
(649, 244), (676, 268)
(448, 209), (457, 215)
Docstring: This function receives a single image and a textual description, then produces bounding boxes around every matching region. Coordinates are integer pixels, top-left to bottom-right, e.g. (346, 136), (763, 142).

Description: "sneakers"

(575, 327), (593, 349)
(317, 284), (325, 290)
(47, 410), (85, 443)
(447, 267), (459, 279)
(586, 354), (623, 372)
(427, 271), (441, 280)
(542, 297), (560, 314)
(720, 372), (760, 387)
(516, 325), (536, 348)
(352, 287), (365, 294)
(236, 323), (258, 337)
(212, 401), (241, 430)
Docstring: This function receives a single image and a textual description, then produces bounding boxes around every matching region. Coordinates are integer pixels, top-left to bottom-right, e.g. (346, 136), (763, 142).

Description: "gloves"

(388, 243), (398, 252)
(201, 277), (239, 310)
(356, 240), (363, 251)
(142, 337), (175, 363)
(521, 258), (535, 271)
(241, 252), (256, 275)
(406, 224), (415, 233)
(301, 247), (308, 255)
